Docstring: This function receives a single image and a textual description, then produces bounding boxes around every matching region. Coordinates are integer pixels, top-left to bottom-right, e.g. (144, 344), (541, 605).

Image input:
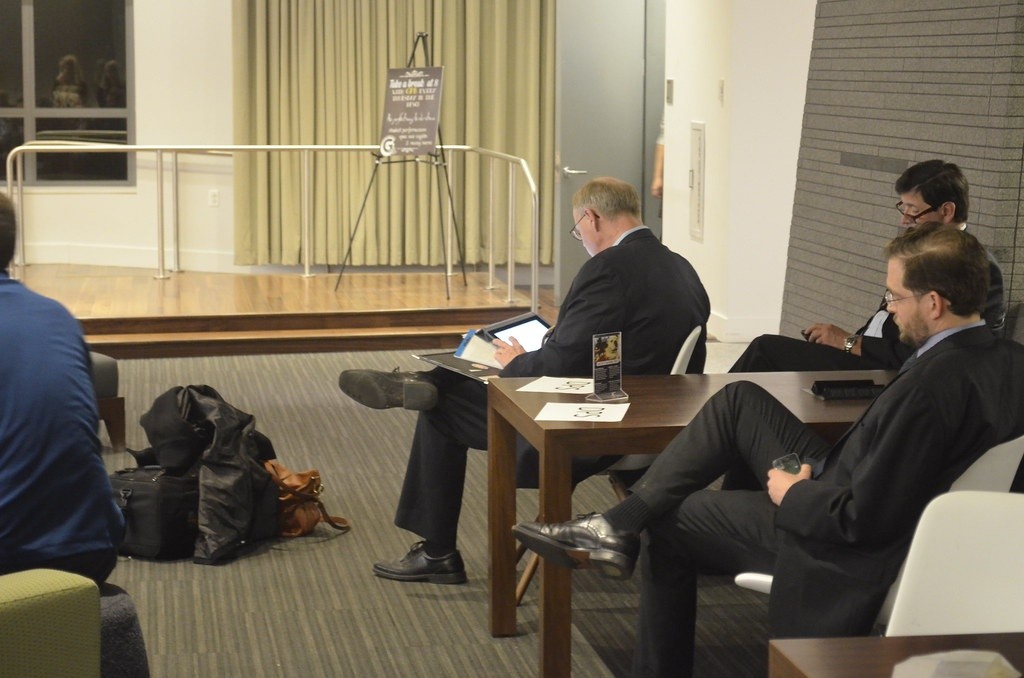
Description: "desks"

(487, 371), (1024, 678)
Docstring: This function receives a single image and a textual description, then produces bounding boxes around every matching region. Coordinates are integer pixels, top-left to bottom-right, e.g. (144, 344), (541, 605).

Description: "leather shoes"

(373, 540), (465, 583)
(339, 366), (438, 411)
(511, 512), (640, 580)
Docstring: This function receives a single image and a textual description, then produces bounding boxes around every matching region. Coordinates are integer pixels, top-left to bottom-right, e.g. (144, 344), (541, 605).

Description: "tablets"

(484, 313), (553, 352)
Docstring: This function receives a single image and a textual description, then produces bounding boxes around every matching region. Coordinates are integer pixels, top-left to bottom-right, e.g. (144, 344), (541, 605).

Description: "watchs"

(845, 334), (858, 352)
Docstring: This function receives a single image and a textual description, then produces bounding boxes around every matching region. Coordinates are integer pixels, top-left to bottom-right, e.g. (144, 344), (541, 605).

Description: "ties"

(898, 350), (917, 375)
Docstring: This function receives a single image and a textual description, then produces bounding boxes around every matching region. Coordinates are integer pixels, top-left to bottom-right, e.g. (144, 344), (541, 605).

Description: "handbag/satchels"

(265, 460), (349, 536)
(110, 466), (199, 561)
(139, 384), (276, 565)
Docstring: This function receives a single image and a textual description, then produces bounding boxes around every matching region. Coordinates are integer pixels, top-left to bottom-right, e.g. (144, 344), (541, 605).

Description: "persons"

(0, 192), (123, 585)
(337, 177), (711, 584)
(509, 223), (1024, 678)
(49, 56), (127, 107)
(726, 160), (1006, 373)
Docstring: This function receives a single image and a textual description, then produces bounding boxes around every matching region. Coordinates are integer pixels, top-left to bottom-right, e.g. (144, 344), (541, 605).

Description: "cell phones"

(773, 453), (801, 475)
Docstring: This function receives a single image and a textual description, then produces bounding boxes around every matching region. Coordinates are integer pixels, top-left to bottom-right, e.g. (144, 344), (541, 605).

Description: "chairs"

(90, 352), (126, 453)
(0, 568), (151, 678)
(734, 435), (1024, 623)
(514, 326), (702, 606)
(886, 491), (1024, 637)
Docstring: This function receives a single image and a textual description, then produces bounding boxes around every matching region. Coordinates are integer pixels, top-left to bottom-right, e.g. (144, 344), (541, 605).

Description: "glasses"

(885, 290), (929, 304)
(569, 213), (600, 241)
(896, 201), (935, 223)
